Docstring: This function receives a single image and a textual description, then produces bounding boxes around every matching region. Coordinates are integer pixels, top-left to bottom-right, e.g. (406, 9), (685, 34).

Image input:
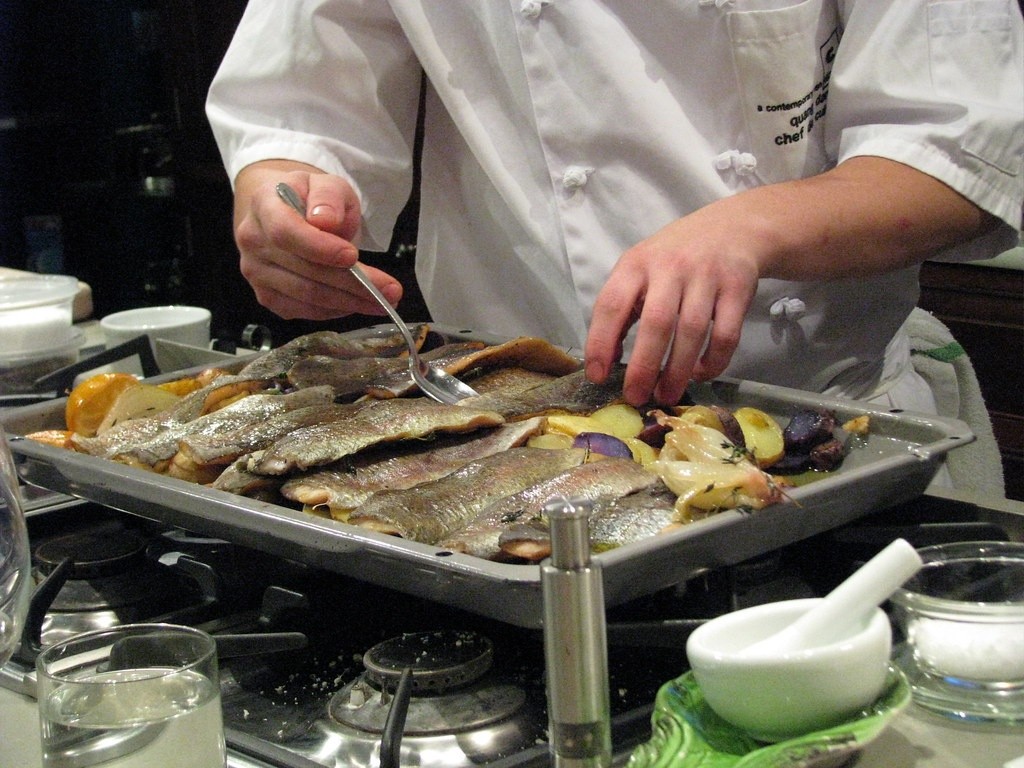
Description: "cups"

(34, 622), (226, 768)
(99, 306), (210, 378)
(0, 423), (32, 673)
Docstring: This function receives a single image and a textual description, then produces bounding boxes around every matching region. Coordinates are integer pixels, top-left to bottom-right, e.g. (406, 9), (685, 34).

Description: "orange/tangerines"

(65, 372), (142, 440)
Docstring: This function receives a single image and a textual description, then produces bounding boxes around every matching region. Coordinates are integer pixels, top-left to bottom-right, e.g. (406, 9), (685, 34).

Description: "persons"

(203, 0), (1023, 493)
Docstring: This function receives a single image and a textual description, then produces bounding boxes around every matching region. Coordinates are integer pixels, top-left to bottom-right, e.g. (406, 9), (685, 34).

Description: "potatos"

(527, 402), (787, 467)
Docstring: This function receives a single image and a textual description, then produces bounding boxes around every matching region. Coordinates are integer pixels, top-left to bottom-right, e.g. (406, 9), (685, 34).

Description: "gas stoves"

(0, 498), (838, 768)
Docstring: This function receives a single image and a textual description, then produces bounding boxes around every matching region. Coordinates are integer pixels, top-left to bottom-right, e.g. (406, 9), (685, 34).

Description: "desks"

(914, 247), (1024, 496)
(0, 316), (1024, 768)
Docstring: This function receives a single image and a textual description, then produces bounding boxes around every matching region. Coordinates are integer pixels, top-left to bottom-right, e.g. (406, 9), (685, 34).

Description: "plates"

(629, 666), (909, 768)
(890, 639), (1024, 725)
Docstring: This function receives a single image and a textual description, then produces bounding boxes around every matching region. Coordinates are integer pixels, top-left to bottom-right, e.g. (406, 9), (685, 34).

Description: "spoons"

(277, 182), (482, 406)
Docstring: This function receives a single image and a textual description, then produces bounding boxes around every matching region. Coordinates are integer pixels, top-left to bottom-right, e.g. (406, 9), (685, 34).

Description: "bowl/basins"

(893, 540), (1024, 697)
(0, 275), (83, 356)
(684, 598), (893, 743)
(0, 326), (87, 397)
